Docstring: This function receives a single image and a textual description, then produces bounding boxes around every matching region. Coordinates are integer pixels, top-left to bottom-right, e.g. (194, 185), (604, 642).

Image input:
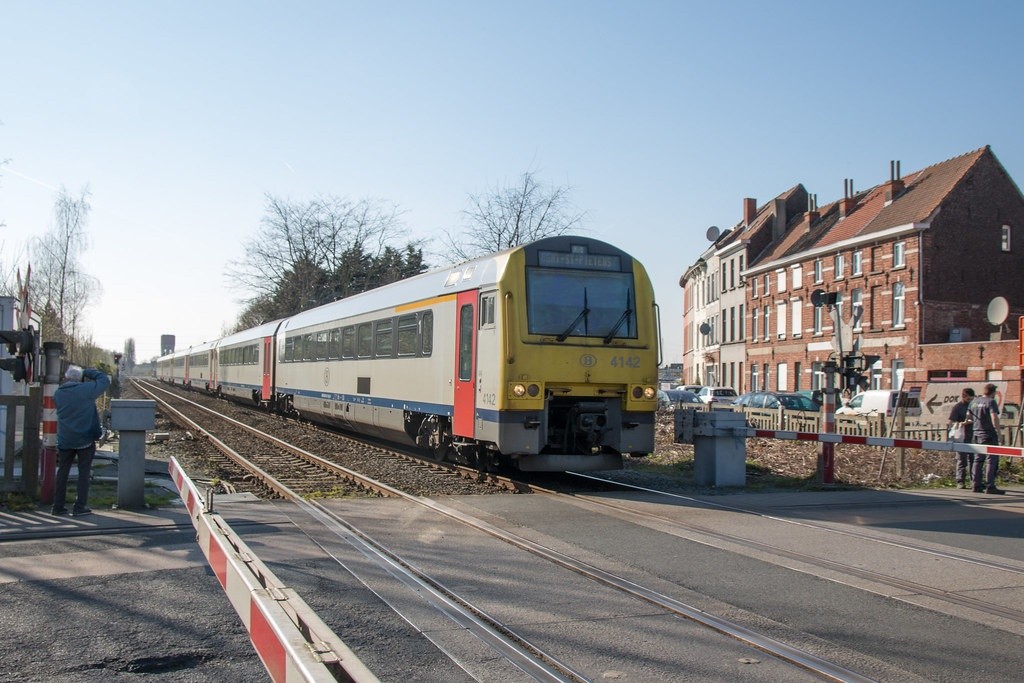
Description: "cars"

(676, 384), (738, 404)
(655, 390), (706, 415)
(730, 389), (843, 430)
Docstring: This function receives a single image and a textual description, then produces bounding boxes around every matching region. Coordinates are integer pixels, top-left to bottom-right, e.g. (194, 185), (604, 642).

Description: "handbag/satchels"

(948, 422), (965, 443)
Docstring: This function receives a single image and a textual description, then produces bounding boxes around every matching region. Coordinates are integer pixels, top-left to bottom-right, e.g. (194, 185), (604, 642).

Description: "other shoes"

(957, 481), (966, 489)
(73, 505), (91, 516)
(973, 486), (986, 492)
(51, 504), (68, 516)
(986, 485), (1005, 495)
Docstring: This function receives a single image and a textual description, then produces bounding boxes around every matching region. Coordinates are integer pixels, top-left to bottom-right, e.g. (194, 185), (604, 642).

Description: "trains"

(156, 234), (663, 473)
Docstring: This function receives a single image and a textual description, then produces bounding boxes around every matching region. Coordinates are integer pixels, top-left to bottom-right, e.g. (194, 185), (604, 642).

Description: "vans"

(836, 390), (906, 422)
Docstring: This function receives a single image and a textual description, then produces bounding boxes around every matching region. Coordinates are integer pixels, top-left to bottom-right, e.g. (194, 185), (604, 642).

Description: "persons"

(948, 383), (1006, 494)
(52, 365), (110, 516)
